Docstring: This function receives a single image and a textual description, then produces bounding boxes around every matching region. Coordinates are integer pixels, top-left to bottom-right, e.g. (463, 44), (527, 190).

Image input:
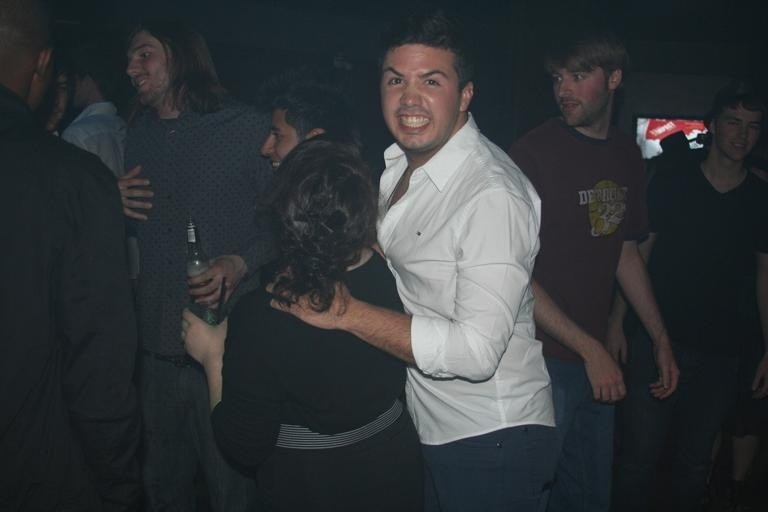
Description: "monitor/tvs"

(633, 112), (710, 161)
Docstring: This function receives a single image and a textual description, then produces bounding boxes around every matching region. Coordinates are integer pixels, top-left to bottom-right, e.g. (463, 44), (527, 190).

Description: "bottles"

(181, 214), (228, 329)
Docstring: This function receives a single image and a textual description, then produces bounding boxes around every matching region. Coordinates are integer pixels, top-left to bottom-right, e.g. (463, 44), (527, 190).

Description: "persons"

(1, 1), (767, 509)
